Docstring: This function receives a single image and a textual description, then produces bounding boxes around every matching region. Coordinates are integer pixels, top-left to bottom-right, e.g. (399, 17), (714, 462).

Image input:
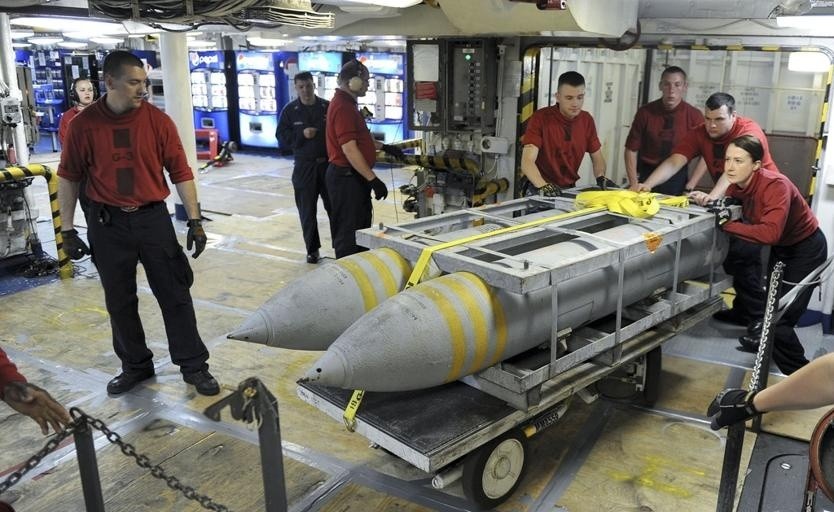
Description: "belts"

(120, 205), (139, 212)
(334, 167), (354, 176)
(295, 156), (328, 163)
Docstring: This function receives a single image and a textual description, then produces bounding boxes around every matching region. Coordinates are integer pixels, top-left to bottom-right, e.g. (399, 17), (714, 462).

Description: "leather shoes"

(306, 254), (319, 263)
(107, 366), (155, 393)
(738, 320), (764, 353)
(183, 368), (220, 396)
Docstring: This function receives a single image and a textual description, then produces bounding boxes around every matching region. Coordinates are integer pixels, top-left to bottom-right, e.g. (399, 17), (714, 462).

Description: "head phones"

(69, 77), (99, 101)
(349, 59), (364, 92)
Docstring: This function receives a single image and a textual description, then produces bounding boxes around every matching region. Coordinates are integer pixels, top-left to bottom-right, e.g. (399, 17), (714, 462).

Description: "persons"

(518, 69), (621, 196)
(705, 350), (833, 432)
(272, 69), (332, 265)
(323, 56), (388, 260)
(57, 77), (99, 151)
(627, 90), (781, 330)
(621, 65), (705, 195)
(685, 133), (829, 378)
(52, 46), (222, 397)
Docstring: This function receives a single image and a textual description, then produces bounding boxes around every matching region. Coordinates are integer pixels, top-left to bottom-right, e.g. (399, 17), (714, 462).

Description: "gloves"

(707, 388), (767, 431)
(707, 208), (732, 228)
(381, 145), (404, 161)
(713, 195), (742, 207)
(186, 220), (207, 258)
(536, 182), (562, 197)
(369, 176), (388, 200)
(60, 229), (90, 260)
(596, 175), (621, 191)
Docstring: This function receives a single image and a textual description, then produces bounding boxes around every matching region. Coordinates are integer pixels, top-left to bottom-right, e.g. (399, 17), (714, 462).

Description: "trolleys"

(292, 293), (725, 512)
